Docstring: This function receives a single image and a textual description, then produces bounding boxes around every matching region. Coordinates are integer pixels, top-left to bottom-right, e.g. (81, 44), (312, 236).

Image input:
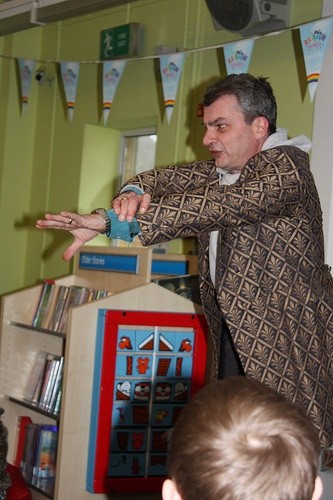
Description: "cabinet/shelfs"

(0, 246), (209, 500)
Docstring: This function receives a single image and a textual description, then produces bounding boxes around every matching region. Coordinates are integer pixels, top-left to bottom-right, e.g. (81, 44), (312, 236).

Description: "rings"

(68, 219), (72, 225)
(120, 196), (129, 202)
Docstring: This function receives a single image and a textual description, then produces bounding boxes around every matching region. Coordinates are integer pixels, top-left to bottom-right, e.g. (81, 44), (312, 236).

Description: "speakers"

(205, 0), (290, 35)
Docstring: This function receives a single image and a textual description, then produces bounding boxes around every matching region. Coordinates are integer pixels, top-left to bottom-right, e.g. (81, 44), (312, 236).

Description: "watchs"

(91, 208), (111, 234)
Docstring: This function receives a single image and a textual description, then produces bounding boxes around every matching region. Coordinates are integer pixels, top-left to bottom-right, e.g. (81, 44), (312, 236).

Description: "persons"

(162, 375), (324, 500)
(35, 73), (333, 472)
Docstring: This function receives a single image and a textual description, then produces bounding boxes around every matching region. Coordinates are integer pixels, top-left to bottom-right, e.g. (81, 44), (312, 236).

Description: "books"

(13, 416), (58, 498)
(22, 351), (65, 415)
(31, 281), (114, 334)
